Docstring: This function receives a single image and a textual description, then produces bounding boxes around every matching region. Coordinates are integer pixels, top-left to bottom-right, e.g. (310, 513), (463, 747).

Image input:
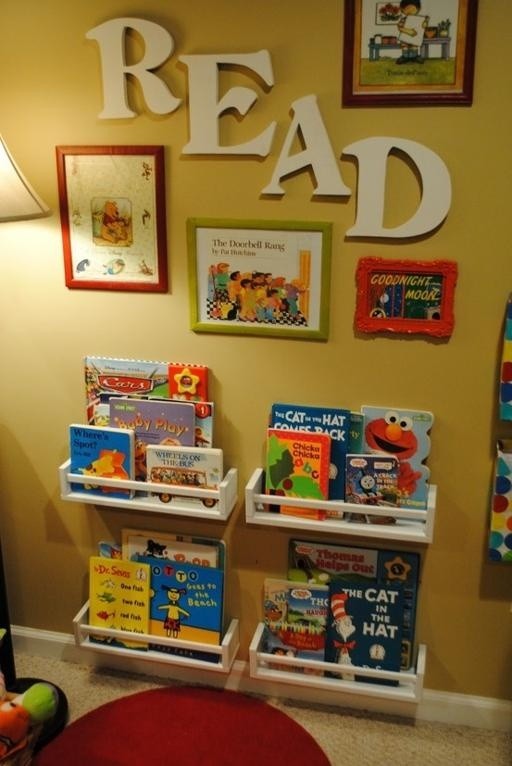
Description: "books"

(68, 358), (226, 514)
(87, 528), (227, 664)
(263, 539), (420, 689)
(265, 403), (434, 524)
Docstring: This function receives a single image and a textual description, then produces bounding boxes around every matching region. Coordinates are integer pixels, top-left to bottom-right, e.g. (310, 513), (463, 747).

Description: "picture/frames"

(338, 0), (479, 105)
(352, 255), (458, 336)
(54, 143), (171, 293)
(187, 215), (334, 340)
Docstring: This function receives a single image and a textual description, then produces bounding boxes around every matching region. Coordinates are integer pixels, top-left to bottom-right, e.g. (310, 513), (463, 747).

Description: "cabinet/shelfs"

(51, 459), (439, 705)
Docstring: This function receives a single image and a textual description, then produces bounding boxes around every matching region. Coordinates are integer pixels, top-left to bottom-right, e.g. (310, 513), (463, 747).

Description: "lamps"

(0, 133), (69, 756)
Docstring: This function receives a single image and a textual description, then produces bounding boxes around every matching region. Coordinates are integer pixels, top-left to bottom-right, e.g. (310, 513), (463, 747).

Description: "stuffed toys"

(0, 668), (60, 760)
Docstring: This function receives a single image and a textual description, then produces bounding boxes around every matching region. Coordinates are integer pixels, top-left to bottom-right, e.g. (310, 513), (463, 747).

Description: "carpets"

(32, 683), (332, 765)
